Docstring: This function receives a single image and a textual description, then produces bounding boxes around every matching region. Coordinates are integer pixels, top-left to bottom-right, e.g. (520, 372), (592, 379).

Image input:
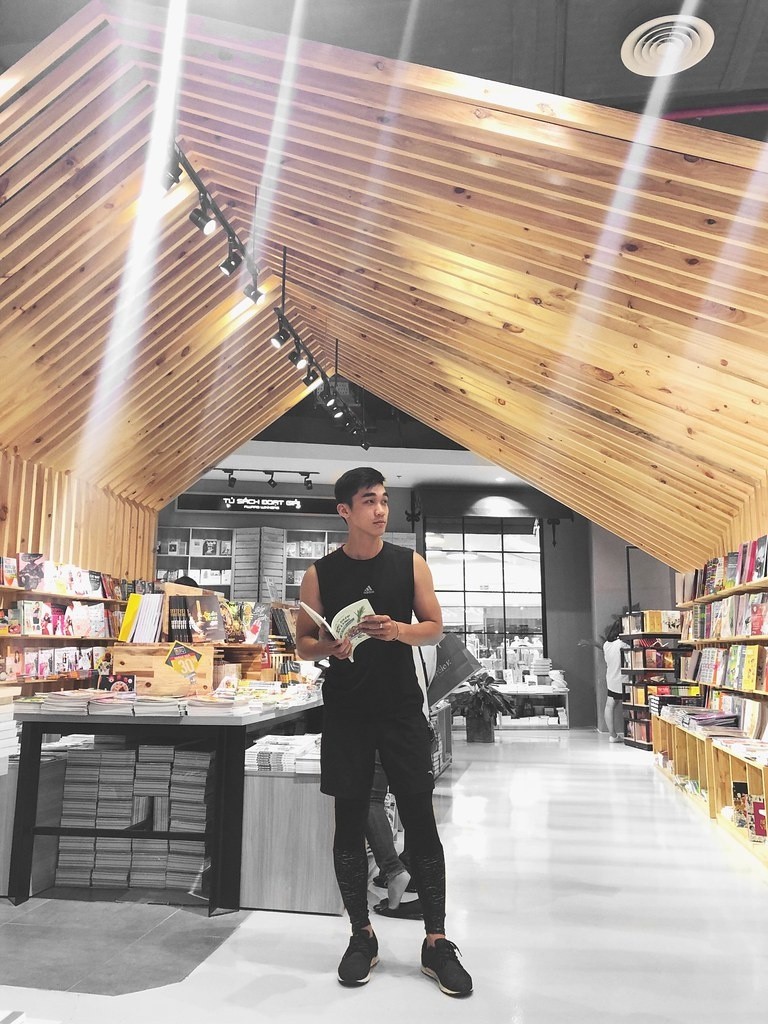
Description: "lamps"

(143, 137), (371, 452)
(212, 468), (320, 489)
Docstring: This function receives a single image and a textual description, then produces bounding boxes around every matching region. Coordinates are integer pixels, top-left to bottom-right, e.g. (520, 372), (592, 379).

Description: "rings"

(379, 622), (383, 629)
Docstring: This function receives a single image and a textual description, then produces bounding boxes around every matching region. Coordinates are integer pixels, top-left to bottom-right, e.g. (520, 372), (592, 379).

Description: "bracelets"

(392, 620), (400, 641)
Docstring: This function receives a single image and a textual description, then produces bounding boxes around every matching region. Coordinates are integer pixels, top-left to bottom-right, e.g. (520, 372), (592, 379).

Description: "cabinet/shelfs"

(0, 583), (128, 684)
(498, 691), (570, 730)
(156, 526), (350, 585)
(0, 686), (22, 776)
(618, 534), (767, 868)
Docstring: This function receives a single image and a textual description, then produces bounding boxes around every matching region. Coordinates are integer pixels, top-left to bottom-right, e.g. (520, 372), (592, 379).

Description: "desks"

(8, 697), (325, 919)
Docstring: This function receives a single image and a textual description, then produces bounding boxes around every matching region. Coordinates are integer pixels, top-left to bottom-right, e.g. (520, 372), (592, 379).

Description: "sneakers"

(337, 929), (380, 986)
(421, 937), (474, 996)
(609, 734), (624, 743)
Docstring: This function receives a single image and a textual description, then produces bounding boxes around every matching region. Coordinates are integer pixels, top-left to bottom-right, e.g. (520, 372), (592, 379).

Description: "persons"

(297, 466), (475, 998)
(365, 609), (437, 920)
(603, 617), (631, 743)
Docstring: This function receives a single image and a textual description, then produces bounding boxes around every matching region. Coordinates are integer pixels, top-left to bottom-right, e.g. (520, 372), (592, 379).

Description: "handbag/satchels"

(427, 630), (482, 708)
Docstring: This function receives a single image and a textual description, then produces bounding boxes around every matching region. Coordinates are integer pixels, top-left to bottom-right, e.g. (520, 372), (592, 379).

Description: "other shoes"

(373, 875), (419, 894)
(373, 898), (424, 920)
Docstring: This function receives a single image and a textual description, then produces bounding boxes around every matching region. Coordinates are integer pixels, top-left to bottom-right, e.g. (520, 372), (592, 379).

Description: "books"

(466, 647), (569, 730)
(9, 734), (220, 892)
(426, 699), (452, 779)
(244, 729), (321, 776)
(158, 536), (339, 558)
(622, 609), (691, 787)
(0, 550), (325, 723)
(0, 707), (21, 757)
(681, 534), (768, 847)
(299, 599), (376, 662)
